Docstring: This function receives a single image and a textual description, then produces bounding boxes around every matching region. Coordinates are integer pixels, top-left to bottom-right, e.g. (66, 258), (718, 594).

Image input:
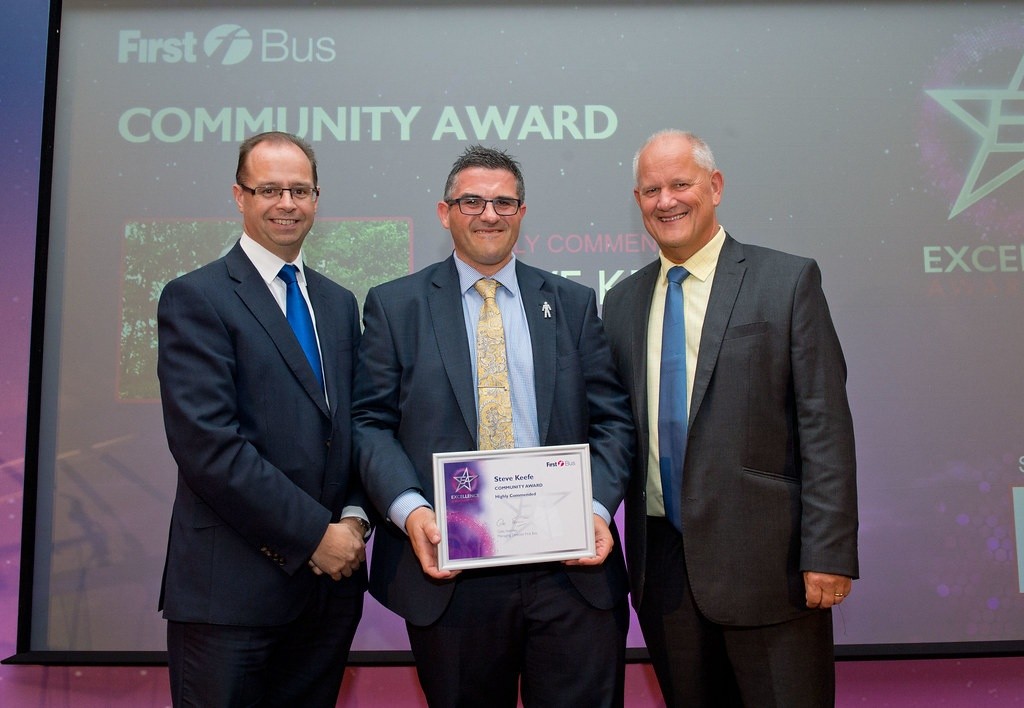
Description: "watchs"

(345, 515), (370, 536)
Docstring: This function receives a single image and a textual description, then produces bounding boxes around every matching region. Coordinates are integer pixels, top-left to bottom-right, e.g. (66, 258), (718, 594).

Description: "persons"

(157, 132), (376, 707)
(352, 146), (640, 708)
(603, 130), (860, 708)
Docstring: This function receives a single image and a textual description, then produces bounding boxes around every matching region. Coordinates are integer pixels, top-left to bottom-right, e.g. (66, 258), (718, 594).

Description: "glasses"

(447, 196), (521, 216)
(239, 184), (320, 201)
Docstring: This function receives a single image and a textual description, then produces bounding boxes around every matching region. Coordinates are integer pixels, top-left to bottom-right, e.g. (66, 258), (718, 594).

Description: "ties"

(657, 267), (687, 534)
(278, 265), (326, 402)
(472, 278), (515, 451)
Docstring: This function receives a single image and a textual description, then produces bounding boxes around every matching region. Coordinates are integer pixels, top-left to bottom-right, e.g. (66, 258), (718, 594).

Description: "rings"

(836, 592), (844, 597)
